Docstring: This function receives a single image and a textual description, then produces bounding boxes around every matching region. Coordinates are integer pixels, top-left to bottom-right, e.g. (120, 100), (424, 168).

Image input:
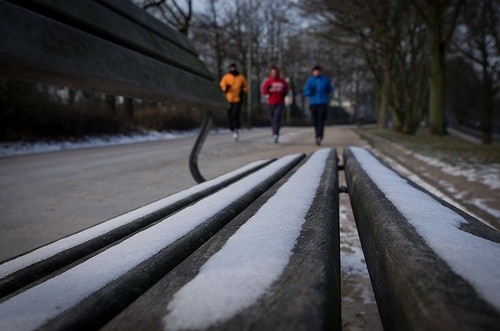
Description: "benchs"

(0, 0), (500, 330)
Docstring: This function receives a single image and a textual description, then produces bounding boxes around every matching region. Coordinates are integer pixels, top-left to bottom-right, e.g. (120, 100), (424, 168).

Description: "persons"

(260, 66), (289, 146)
(219, 61), (248, 141)
(302, 66), (333, 145)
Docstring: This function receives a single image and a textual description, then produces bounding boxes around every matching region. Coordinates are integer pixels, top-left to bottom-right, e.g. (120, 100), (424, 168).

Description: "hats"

(228, 63), (237, 68)
(312, 64), (322, 72)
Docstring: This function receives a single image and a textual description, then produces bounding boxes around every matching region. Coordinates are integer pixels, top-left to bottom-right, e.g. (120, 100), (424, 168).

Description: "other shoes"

(272, 133), (280, 144)
(314, 136), (322, 146)
(232, 129), (239, 142)
